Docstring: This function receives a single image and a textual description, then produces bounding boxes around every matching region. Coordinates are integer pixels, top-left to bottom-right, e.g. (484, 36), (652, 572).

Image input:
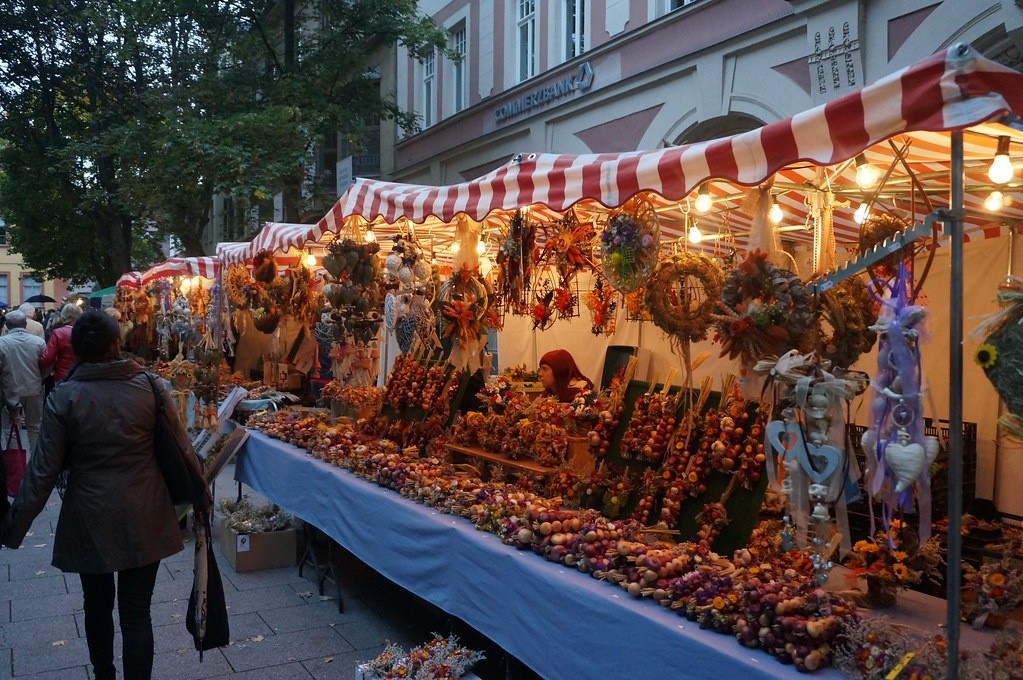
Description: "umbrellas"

(23, 293), (57, 319)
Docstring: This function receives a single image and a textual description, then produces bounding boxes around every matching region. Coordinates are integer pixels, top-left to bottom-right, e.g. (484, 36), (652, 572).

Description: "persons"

(535, 348), (597, 431)
(0, 296), (102, 492)
(0, 310), (213, 680)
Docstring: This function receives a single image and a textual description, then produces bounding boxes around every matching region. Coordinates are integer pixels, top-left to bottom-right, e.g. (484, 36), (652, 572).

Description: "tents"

(113, 42), (1022, 680)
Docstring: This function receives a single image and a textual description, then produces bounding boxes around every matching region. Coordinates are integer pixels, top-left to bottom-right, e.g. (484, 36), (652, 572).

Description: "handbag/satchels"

(184, 511), (229, 662)
(3, 420), (26, 497)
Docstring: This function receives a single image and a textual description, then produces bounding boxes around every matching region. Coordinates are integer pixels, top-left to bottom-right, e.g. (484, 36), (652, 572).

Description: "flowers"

(113, 206), (1023, 679)
